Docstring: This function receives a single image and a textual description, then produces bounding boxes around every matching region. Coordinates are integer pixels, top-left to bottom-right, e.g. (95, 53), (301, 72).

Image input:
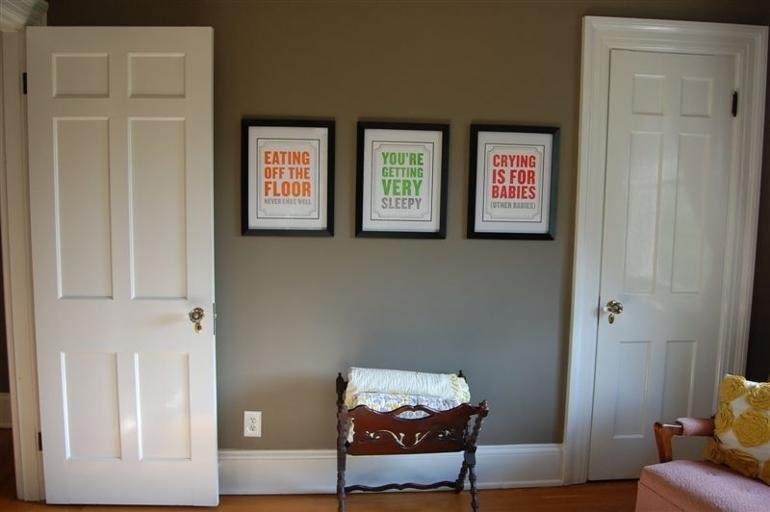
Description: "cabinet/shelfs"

(332, 368), (490, 511)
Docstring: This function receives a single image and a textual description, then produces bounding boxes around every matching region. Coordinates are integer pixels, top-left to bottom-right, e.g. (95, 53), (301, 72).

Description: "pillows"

(705, 372), (770, 485)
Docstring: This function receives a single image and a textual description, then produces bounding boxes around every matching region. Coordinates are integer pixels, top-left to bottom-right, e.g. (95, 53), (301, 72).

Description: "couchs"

(634, 409), (770, 512)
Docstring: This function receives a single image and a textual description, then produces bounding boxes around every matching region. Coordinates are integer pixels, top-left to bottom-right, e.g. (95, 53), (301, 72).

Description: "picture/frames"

(240, 117), (336, 237)
(354, 120), (451, 240)
(466, 122), (559, 240)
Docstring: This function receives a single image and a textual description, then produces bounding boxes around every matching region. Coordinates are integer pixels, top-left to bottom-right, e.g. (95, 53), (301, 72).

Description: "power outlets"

(244, 410), (262, 437)
